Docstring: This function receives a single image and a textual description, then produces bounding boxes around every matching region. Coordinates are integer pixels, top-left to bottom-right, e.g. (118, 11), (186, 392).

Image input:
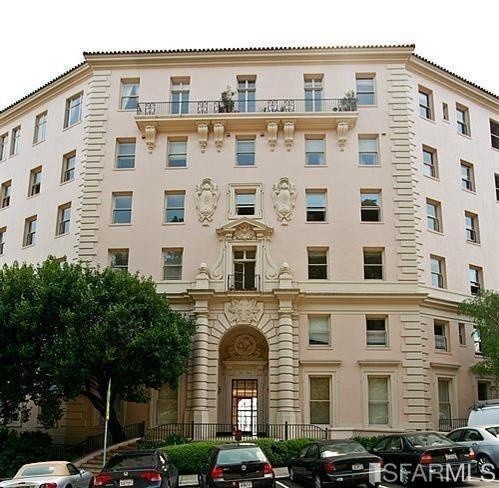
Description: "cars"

(0, 461), (95, 488)
(286, 399), (499, 488)
(197, 444), (276, 488)
(93, 449), (179, 488)
